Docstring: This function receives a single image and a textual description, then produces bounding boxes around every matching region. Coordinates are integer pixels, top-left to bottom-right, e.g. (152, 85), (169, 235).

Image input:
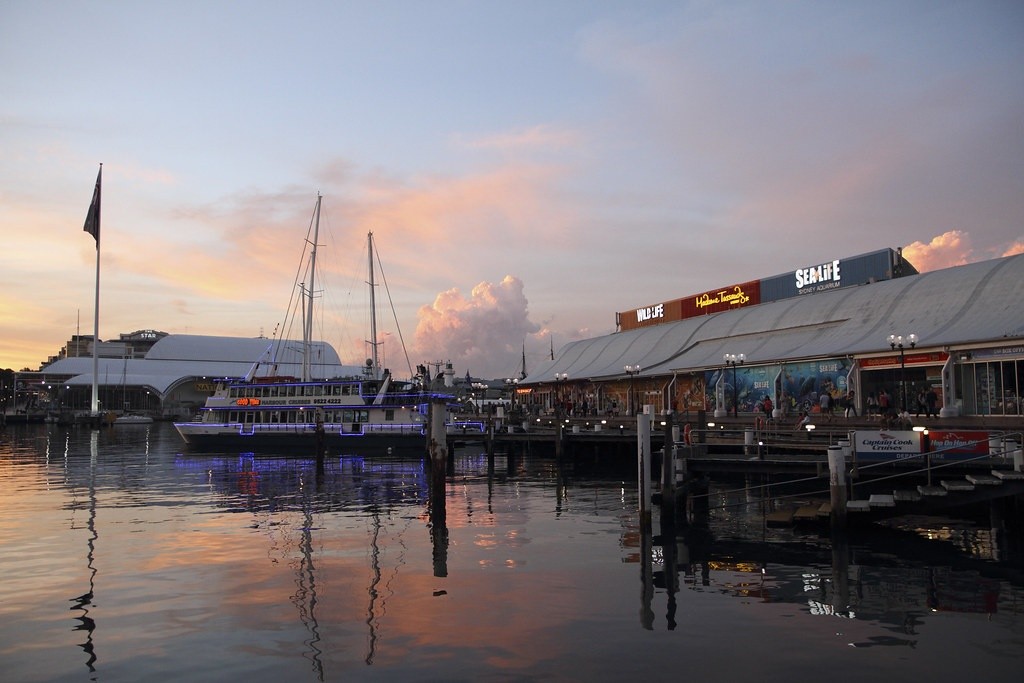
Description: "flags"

(83, 162), (103, 247)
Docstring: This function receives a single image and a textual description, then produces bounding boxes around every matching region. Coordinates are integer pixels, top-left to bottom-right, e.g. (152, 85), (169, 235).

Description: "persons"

(762, 384), (940, 433)
(782, 373), (798, 407)
(565, 397), (620, 419)
(460, 401), (544, 419)
(315, 410), (320, 423)
(671, 393), (689, 421)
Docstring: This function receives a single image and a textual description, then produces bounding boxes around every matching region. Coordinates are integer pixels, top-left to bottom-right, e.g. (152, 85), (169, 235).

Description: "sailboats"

(112, 354), (153, 423)
(172, 190), (489, 449)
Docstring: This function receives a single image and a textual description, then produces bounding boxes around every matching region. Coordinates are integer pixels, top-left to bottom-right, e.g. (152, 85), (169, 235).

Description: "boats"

(29, 416), (59, 423)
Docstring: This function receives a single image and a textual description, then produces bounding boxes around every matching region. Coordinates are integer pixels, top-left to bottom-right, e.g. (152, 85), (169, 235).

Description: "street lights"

(554, 372), (567, 416)
(723, 353), (746, 418)
(506, 379), (519, 411)
(886, 333), (919, 412)
(458, 382), (488, 415)
(623, 365), (641, 416)
